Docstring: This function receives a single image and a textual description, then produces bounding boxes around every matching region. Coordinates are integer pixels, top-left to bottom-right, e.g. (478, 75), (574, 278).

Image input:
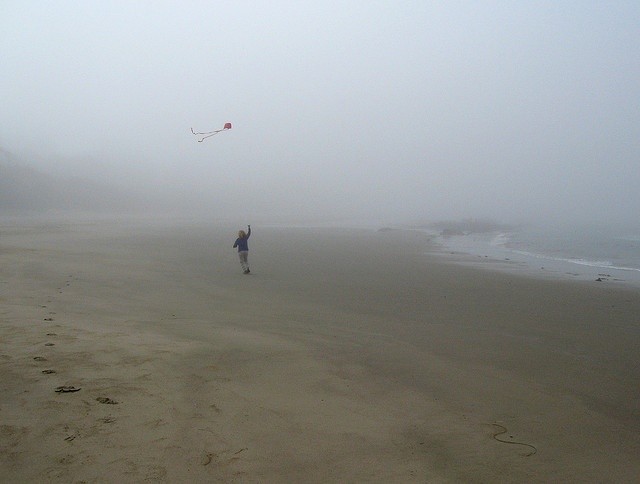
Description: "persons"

(233, 225), (252, 274)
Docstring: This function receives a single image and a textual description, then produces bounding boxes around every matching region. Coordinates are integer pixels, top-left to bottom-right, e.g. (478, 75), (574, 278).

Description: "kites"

(190, 122), (232, 143)
